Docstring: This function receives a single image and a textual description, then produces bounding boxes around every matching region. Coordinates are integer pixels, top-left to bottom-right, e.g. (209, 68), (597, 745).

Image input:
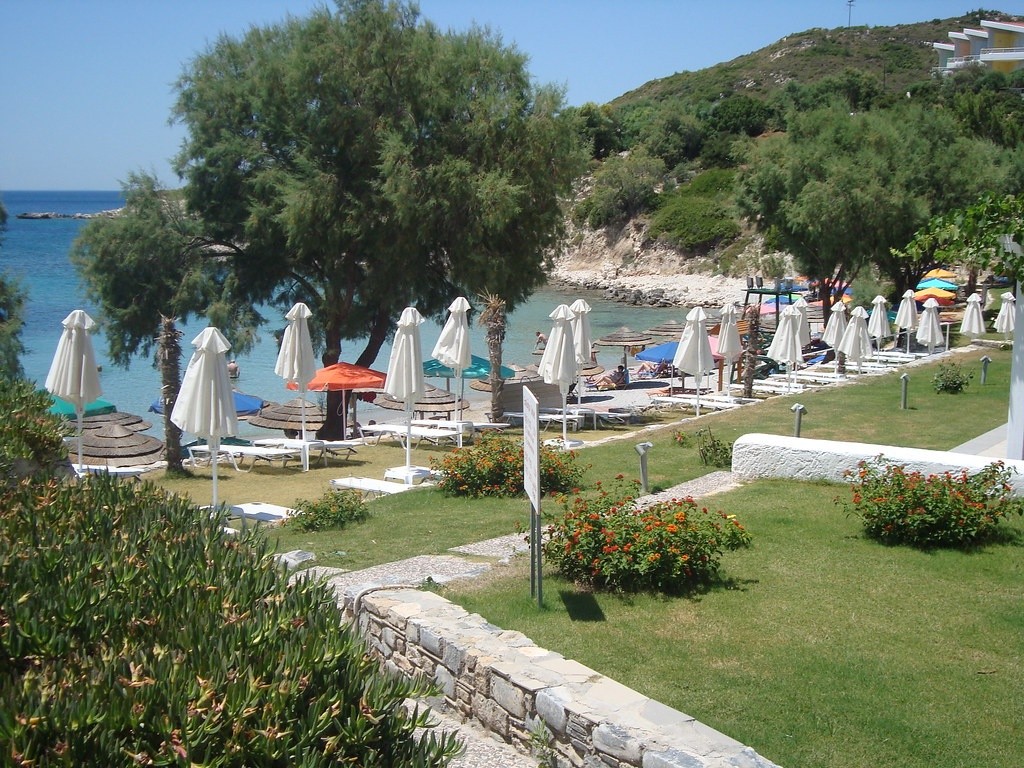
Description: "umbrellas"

(672, 307), (714, 417)
(383, 307), (426, 483)
(792, 297), (812, 380)
(914, 268), (957, 306)
(44, 310), (105, 472)
(538, 304), (578, 439)
(821, 289), (1016, 375)
(635, 341), (678, 377)
(743, 276), (854, 338)
(286, 361), (387, 440)
(423, 354), (516, 420)
(637, 362), (665, 373)
(716, 304), (742, 395)
(169, 327), (239, 506)
(274, 303), (317, 472)
(431, 297), (472, 419)
(767, 304), (804, 392)
(569, 298), (592, 409)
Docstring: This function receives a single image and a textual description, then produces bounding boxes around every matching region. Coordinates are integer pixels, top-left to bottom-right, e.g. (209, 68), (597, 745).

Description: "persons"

(592, 365), (623, 385)
(534, 331), (548, 350)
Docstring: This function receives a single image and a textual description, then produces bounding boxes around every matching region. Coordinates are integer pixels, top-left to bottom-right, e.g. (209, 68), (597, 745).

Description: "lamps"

(790, 402), (807, 415)
(634, 442), (653, 456)
(900, 372), (911, 383)
(980, 356), (991, 364)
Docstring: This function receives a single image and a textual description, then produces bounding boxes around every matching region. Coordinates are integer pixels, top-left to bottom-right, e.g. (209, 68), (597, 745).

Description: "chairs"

(71, 350), (928, 537)
